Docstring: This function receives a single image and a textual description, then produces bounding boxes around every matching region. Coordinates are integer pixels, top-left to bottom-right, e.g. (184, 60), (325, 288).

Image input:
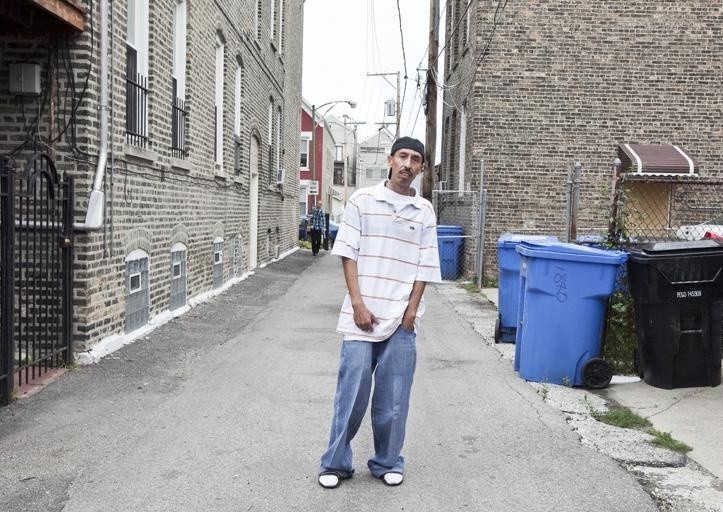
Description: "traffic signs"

(309, 181), (318, 195)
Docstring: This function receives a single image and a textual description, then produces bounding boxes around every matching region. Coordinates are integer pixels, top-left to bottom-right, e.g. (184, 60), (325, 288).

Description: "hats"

(388, 137), (424, 180)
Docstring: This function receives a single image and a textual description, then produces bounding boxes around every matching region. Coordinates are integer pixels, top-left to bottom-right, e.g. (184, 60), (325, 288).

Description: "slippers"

(319, 471), (341, 488)
(380, 472), (404, 485)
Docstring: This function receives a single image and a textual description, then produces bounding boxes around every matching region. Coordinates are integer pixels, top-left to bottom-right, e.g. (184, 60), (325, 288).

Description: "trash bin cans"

(437, 225), (465, 280)
(494, 231), (723, 388)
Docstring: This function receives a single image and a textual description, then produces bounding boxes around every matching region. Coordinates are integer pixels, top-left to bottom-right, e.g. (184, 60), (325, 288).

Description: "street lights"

(312, 98), (358, 207)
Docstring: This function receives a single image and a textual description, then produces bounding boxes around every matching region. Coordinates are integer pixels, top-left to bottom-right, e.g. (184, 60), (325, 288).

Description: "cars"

(677, 213), (723, 244)
(299, 216), (339, 251)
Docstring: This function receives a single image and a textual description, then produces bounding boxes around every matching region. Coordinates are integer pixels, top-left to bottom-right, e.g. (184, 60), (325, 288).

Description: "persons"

(309, 200), (326, 256)
(318, 136), (442, 488)
